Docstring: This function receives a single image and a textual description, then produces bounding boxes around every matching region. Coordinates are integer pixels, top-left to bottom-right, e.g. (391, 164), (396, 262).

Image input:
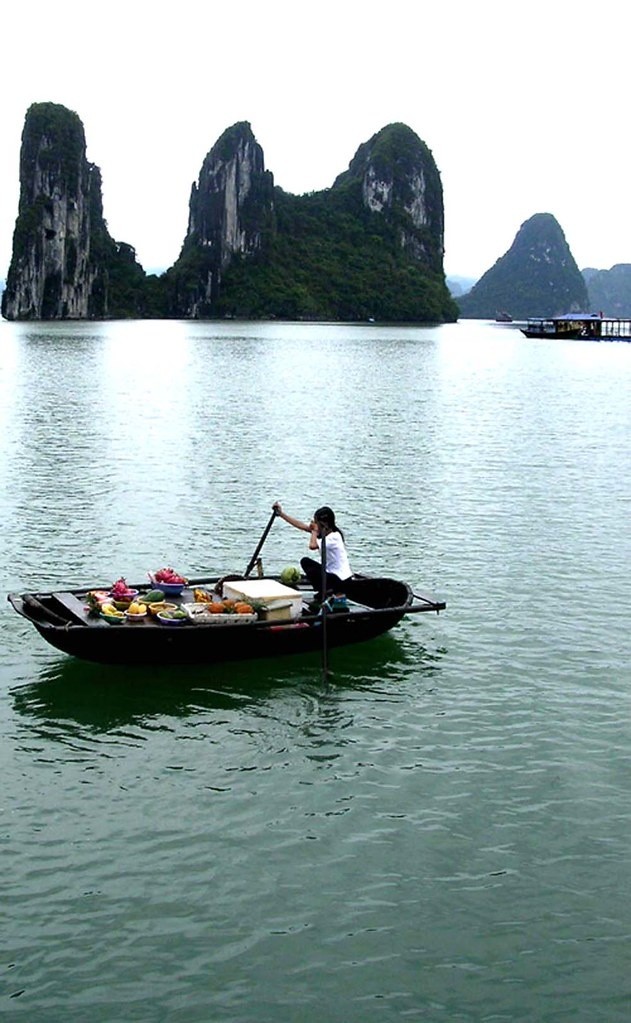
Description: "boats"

(518, 311), (631, 341)
(496, 311), (513, 322)
(5, 558), (448, 671)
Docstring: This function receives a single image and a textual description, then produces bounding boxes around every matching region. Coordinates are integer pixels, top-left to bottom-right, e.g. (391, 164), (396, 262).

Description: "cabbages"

(281, 566), (301, 584)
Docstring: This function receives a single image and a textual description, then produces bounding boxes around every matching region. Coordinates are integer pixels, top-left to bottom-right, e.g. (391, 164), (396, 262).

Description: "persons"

(271, 501), (354, 600)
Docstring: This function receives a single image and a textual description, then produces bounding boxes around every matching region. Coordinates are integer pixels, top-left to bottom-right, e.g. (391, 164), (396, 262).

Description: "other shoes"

(314, 592), (322, 599)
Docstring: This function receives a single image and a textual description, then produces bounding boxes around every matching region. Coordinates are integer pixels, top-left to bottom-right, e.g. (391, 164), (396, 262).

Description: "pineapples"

(207, 599), (254, 614)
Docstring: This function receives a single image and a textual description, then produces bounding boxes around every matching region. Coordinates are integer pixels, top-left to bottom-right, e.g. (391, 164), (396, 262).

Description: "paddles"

(243, 507), (277, 577)
(318, 520), (329, 671)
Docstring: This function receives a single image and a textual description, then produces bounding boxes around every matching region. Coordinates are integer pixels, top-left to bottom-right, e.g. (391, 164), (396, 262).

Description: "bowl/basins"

(150, 582), (184, 594)
(117, 589), (139, 600)
(124, 609), (147, 621)
(137, 597), (165, 605)
(157, 611), (186, 625)
(148, 603), (178, 615)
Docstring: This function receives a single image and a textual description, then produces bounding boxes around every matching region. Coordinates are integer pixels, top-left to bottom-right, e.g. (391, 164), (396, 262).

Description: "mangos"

(129, 602), (147, 614)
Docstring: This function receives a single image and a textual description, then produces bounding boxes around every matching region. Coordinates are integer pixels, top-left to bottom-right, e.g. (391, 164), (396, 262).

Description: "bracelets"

(312, 529), (318, 531)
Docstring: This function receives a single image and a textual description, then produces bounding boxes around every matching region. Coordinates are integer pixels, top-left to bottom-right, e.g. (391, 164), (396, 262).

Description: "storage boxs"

(181, 601), (258, 623)
(223, 578), (302, 619)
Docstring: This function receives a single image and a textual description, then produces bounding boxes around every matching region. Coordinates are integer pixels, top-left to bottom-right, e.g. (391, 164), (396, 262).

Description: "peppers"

(160, 611), (184, 619)
